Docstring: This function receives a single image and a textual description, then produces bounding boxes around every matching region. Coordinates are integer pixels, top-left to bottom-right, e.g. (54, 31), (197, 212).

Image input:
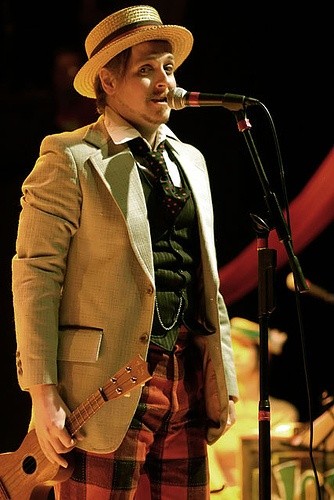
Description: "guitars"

(0, 353), (154, 500)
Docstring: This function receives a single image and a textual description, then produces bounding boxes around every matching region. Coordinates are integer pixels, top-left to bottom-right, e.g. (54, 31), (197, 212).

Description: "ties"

(132, 139), (190, 226)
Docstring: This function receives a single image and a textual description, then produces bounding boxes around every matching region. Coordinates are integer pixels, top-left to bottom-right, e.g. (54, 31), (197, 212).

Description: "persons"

(11, 6), (241, 500)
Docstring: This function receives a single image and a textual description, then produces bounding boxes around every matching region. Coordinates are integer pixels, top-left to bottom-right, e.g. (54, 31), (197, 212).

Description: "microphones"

(168, 87), (259, 110)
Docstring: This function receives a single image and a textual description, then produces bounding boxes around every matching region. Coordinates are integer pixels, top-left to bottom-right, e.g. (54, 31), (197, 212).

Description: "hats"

(73, 5), (195, 98)
